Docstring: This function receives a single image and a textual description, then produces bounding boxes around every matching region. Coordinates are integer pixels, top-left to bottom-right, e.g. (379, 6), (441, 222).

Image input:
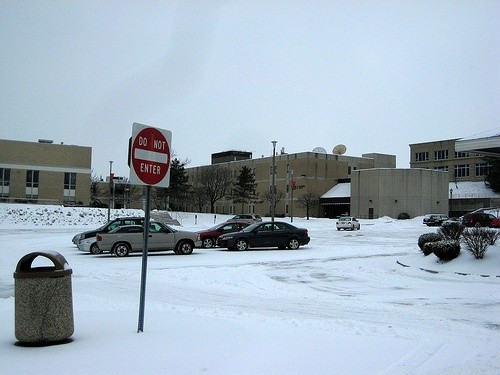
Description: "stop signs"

(127, 122), (173, 188)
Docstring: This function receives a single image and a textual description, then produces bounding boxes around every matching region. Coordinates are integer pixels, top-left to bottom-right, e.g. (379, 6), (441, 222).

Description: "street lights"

(272, 141), (278, 223)
(107, 161), (114, 223)
(290, 169), (294, 223)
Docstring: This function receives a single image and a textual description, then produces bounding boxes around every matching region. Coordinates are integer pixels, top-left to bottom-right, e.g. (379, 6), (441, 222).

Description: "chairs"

(269, 225), (276, 229)
(265, 226), (269, 230)
(151, 225), (155, 229)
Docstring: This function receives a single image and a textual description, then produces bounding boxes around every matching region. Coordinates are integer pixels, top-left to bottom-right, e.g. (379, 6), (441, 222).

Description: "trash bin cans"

(13, 250), (74, 343)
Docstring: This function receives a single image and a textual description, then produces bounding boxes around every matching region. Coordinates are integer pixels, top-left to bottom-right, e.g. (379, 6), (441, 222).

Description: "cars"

(422, 213), (500, 228)
(197, 214), (310, 251)
(336, 217), (361, 231)
(71, 216), (201, 258)
